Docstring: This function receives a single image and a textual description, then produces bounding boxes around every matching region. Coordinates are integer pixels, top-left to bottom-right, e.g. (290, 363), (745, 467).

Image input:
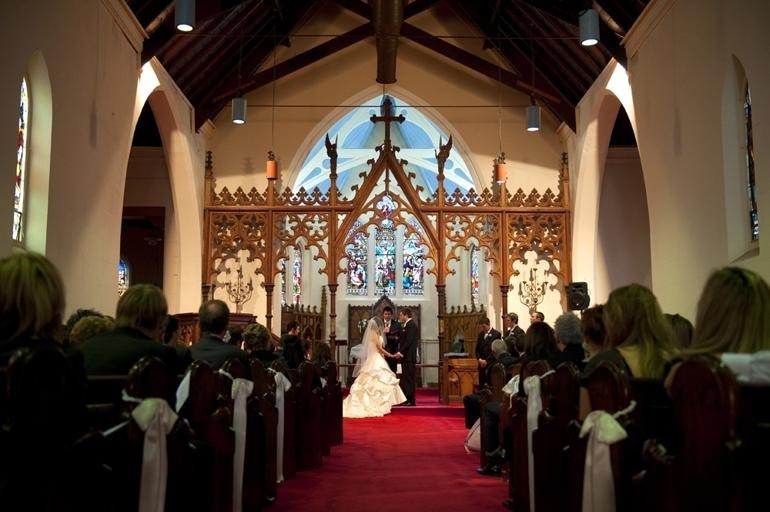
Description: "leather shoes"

(402, 402), (415, 406)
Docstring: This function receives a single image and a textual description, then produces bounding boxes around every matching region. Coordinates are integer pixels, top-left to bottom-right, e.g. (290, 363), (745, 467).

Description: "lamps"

(578, 2), (600, 47)
(496, 54), (507, 185)
(232, 36), (248, 125)
(266, 47), (278, 181)
(174, 0), (196, 33)
(526, 25), (541, 133)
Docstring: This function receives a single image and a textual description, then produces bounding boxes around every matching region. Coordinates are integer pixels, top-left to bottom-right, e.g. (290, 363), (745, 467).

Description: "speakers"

(568, 282), (590, 310)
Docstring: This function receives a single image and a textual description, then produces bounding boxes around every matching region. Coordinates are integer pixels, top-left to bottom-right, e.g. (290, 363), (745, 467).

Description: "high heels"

(478, 457), (504, 475)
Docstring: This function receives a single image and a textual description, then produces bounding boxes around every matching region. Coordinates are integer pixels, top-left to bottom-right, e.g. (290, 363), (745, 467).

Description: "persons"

(661, 266), (770, 452)
(65, 283), (335, 418)
(381, 307), (400, 373)
(345, 250), (423, 297)
(395, 309), (418, 405)
(463, 282), (692, 511)
(343, 315), (408, 419)
(2, 251), (101, 505)
